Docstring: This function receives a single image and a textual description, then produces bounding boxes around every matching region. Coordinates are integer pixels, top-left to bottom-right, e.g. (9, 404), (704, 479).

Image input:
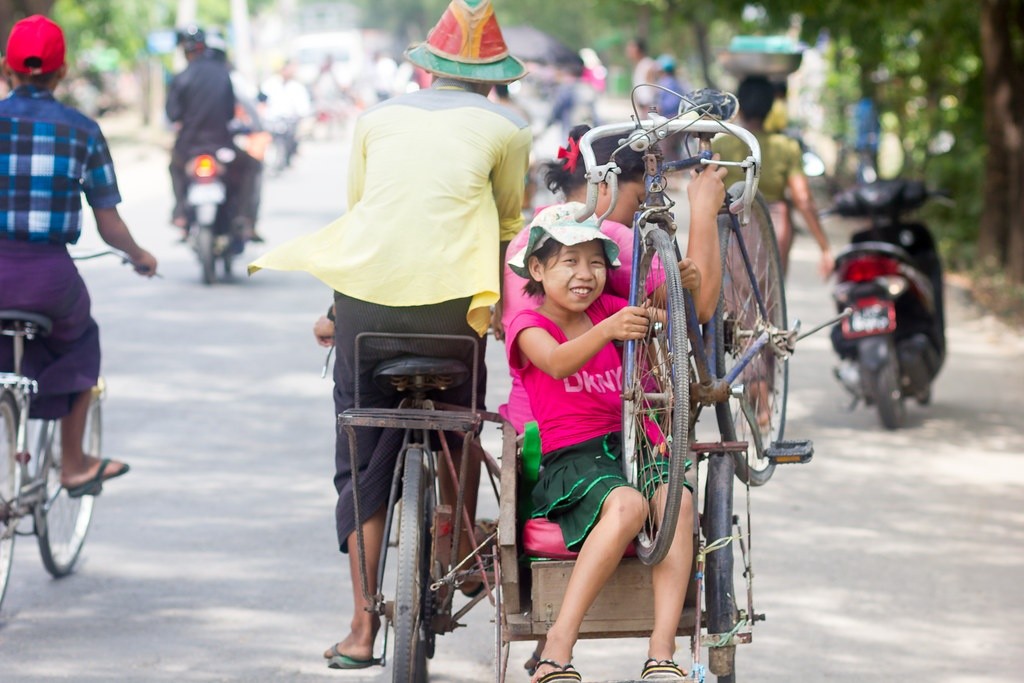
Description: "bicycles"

(572, 82), (855, 568)
(0, 249), (168, 612)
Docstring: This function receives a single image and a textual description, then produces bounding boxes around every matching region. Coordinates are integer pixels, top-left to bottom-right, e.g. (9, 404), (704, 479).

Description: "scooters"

(801, 130), (956, 433)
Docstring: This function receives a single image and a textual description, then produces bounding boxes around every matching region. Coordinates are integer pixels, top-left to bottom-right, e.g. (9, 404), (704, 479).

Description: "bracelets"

(326, 305), (335, 322)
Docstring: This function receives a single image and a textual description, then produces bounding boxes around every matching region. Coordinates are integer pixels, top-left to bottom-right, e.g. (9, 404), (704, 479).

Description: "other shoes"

(243, 233), (263, 242)
(172, 226), (189, 241)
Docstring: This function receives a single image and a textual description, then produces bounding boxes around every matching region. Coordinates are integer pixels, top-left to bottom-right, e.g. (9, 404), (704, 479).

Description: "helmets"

(205, 30), (225, 52)
(176, 27), (202, 45)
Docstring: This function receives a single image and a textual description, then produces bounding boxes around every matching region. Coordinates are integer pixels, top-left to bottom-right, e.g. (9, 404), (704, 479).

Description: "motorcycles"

(167, 88), (272, 284)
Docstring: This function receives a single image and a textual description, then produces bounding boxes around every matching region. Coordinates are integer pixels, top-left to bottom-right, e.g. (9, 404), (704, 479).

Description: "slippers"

(327, 644), (374, 668)
(465, 519), (494, 598)
(642, 659), (684, 679)
(535, 659), (582, 683)
(69, 460), (130, 498)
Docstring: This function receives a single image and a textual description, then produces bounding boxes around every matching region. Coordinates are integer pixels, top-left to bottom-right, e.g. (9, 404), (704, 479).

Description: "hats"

(6, 15), (65, 75)
(654, 55), (677, 72)
(506, 202), (621, 277)
(404, 0), (529, 82)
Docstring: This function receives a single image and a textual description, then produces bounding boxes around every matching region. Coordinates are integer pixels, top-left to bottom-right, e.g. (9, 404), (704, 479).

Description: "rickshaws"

(317, 329), (767, 683)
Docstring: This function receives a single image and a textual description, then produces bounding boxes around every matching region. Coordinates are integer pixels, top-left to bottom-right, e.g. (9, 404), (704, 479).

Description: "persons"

(0, 14), (158, 489)
(499, 124), (730, 437)
(166, 26), (265, 241)
(506, 202), (694, 683)
(245, 0), (535, 670)
(525, 48), (608, 146)
(711, 73), (834, 436)
(624, 36), (678, 118)
